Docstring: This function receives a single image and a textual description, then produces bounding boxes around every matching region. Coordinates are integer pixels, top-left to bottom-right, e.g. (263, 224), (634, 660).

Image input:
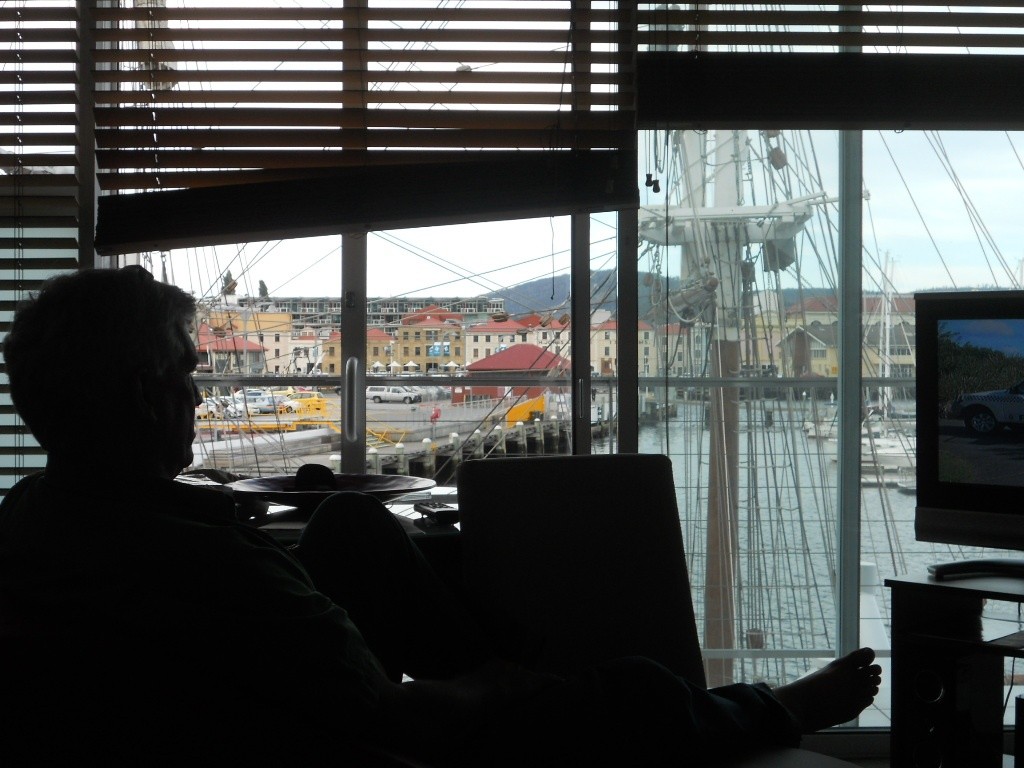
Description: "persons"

(0, 263), (883, 767)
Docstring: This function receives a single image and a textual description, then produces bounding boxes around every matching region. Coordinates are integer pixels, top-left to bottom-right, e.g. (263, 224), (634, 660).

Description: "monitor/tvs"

(914, 290), (1024, 553)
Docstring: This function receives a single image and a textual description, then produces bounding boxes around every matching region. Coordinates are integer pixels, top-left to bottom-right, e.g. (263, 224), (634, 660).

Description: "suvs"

(367, 385), (462, 404)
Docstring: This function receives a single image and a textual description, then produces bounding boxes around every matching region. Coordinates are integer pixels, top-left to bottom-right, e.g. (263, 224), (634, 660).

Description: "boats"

(638, 3), (920, 686)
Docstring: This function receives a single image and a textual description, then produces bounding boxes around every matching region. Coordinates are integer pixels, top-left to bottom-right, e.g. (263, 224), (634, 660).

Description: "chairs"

(456, 452), (866, 768)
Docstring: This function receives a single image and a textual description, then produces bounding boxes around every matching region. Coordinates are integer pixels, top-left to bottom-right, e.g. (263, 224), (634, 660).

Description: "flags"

(505, 396), (548, 431)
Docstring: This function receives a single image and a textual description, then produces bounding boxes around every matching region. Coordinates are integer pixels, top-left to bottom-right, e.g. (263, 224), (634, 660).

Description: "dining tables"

(237, 486), (459, 544)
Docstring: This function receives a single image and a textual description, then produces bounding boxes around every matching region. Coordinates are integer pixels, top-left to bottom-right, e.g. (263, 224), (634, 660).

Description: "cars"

(200, 389), (327, 416)
(952, 376), (1024, 436)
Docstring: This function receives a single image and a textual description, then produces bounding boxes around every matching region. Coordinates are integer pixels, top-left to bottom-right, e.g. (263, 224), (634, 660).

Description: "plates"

(220, 471), (439, 510)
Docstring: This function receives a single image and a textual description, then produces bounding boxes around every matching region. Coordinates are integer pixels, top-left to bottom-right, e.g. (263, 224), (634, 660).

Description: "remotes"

(413, 500), (460, 524)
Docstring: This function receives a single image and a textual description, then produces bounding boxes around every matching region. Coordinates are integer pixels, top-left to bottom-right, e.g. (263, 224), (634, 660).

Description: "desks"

(883, 574), (1024, 768)
